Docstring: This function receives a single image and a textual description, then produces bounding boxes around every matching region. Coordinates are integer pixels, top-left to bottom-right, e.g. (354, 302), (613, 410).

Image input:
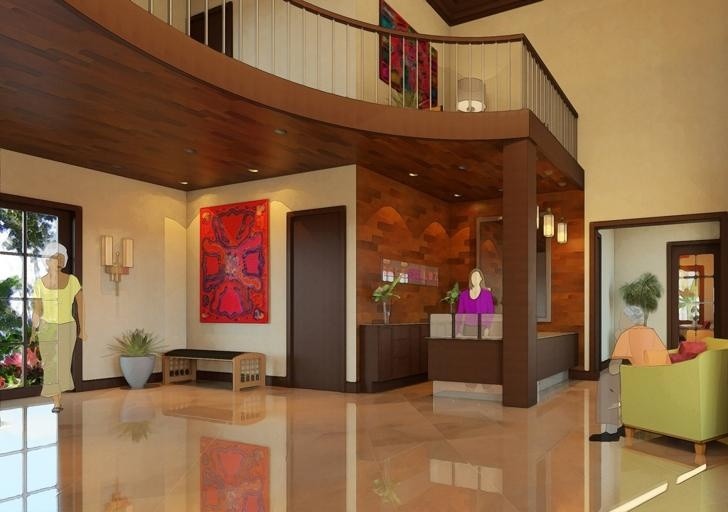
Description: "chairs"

(620, 336), (728, 453)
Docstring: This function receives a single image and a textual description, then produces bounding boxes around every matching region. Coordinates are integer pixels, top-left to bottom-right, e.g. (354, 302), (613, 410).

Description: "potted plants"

(102, 326), (168, 389)
(372, 273), (403, 325)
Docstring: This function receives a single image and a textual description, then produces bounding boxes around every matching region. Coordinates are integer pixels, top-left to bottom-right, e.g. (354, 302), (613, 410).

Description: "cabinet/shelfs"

(360, 322), (429, 384)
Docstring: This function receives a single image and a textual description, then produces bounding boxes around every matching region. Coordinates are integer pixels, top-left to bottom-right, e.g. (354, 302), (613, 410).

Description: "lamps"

(99, 235), (135, 298)
(543, 171), (569, 244)
(456, 77), (488, 112)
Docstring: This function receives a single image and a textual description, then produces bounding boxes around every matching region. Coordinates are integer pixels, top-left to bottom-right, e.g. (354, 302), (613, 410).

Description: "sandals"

(51, 404), (64, 414)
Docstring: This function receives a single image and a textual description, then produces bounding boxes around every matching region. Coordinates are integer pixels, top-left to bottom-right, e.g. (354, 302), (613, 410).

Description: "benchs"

(162, 348), (266, 390)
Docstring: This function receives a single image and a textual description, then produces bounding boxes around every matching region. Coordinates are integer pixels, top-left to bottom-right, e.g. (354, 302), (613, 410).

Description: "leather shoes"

(590, 425), (625, 442)
